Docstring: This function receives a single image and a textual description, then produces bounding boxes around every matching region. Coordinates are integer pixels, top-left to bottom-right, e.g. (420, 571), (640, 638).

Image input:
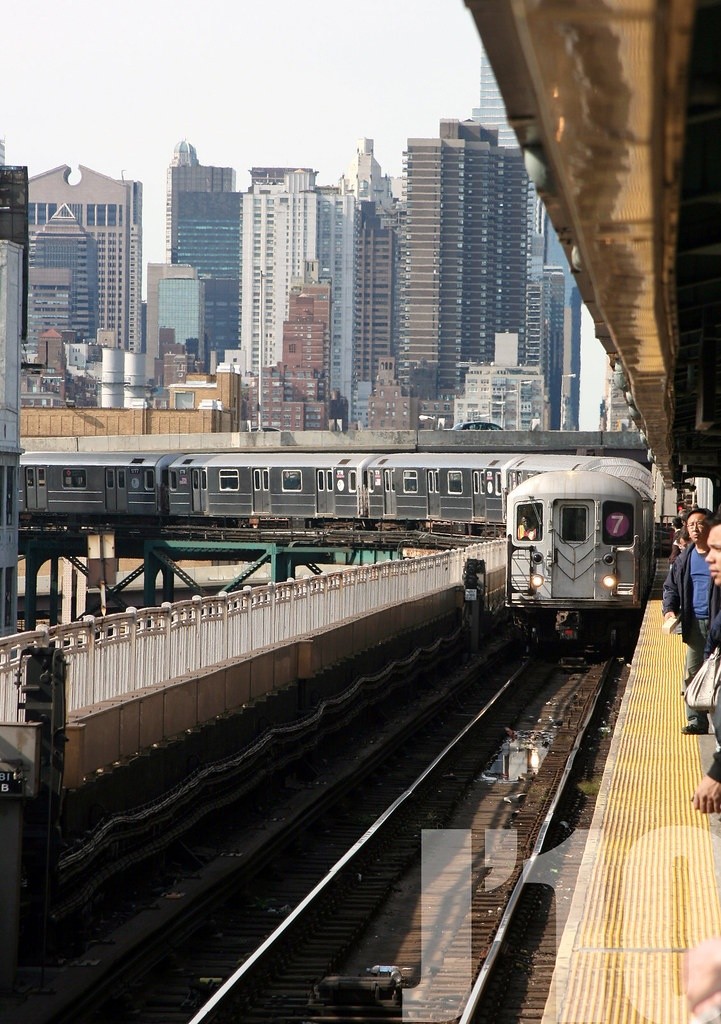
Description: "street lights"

(488, 399), (506, 423)
(518, 380), (533, 431)
(500, 388), (518, 428)
(561, 374), (576, 432)
(257, 270), (268, 431)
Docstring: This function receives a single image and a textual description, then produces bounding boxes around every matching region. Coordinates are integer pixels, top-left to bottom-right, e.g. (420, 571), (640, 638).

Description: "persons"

(516, 516), (536, 540)
(682, 938), (721, 1024)
(662, 497), (721, 823)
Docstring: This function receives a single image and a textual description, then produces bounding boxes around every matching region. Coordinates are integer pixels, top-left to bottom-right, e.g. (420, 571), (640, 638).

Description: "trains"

(17, 449), (657, 673)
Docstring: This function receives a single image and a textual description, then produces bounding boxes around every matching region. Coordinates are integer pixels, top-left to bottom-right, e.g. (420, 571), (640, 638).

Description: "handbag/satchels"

(684, 648), (721, 710)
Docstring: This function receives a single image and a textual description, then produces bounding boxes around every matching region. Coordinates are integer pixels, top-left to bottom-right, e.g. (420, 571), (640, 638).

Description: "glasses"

(687, 522), (707, 529)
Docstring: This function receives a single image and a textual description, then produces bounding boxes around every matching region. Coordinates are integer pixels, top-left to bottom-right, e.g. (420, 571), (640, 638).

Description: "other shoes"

(681, 724), (708, 735)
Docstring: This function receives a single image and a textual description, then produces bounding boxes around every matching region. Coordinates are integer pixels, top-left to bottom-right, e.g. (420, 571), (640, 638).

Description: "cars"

(452, 420), (505, 430)
(244, 425), (281, 432)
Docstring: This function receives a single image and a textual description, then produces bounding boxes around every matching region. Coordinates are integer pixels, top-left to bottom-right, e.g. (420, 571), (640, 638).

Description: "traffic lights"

(676, 498), (684, 514)
